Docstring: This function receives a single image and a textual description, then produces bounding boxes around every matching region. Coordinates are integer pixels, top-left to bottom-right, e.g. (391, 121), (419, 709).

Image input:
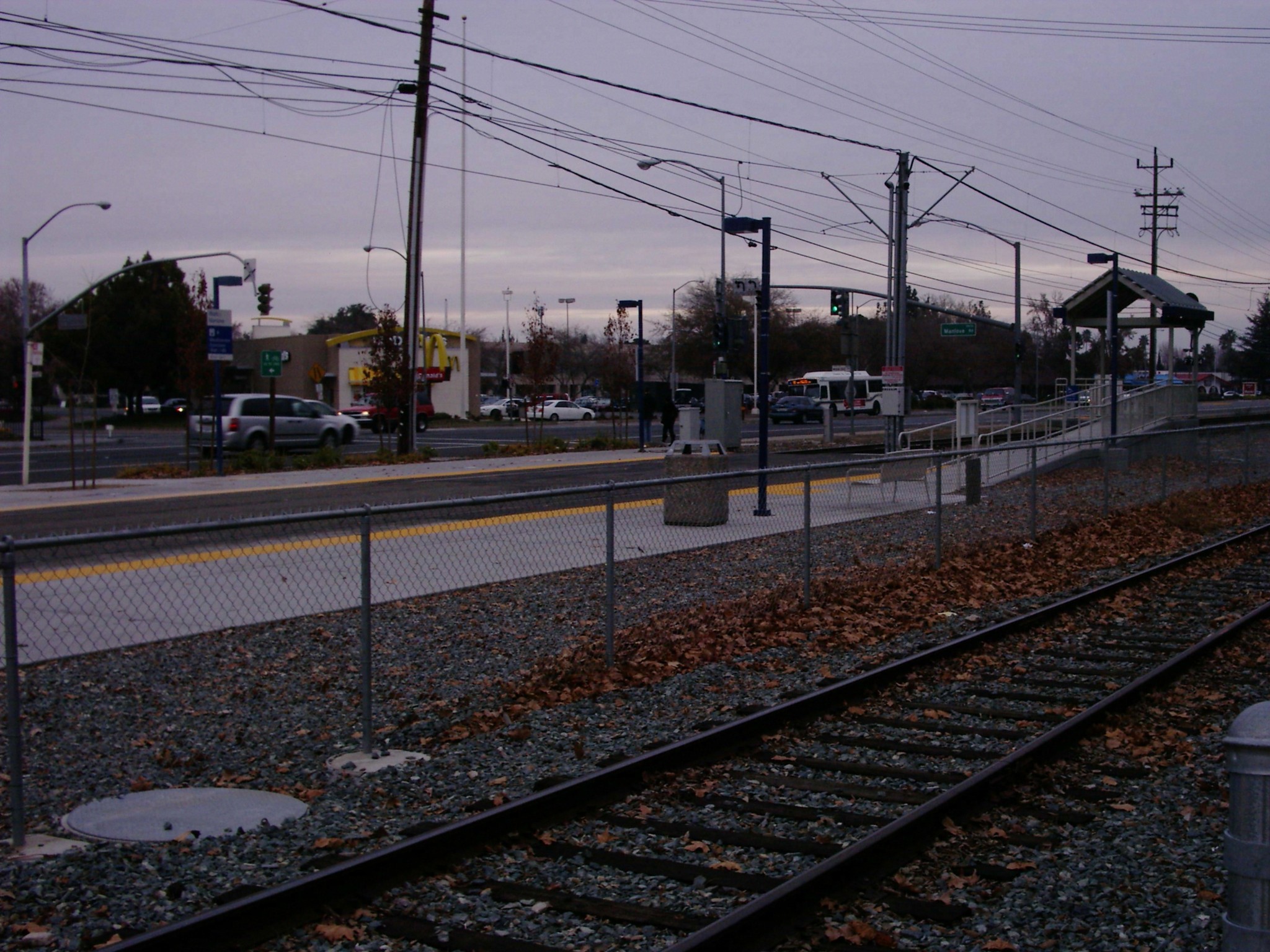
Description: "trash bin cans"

(662, 440), (730, 527)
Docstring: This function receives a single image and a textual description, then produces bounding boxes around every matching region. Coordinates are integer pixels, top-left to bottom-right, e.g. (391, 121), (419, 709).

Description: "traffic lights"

(831, 290), (847, 316)
(258, 283), (274, 316)
(1015, 342), (1023, 359)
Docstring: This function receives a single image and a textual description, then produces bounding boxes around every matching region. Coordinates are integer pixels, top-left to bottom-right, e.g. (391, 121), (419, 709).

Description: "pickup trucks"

(339, 392), (436, 434)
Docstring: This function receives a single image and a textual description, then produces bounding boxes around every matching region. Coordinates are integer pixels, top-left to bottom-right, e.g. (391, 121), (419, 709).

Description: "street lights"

(502, 286), (513, 399)
(1087, 252), (1118, 446)
(636, 157), (725, 378)
(618, 299), (647, 453)
(22, 200), (111, 482)
(558, 298), (576, 396)
(722, 215), (772, 517)
(363, 245), (429, 404)
(671, 280), (705, 404)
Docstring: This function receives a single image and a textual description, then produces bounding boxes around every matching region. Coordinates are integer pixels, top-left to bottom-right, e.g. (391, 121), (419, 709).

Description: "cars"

(1078, 390), (1090, 405)
(162, 398), (187, 417)
(125, 396), (161, 419)
(745, 369), (975, 427)
(479, 392), (631, 424)
(304, 399), (360, 446)
(1224, 391), (1242, 397)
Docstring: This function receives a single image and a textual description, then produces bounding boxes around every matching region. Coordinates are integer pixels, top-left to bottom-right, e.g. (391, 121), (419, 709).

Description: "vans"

(980, 387), (1016, 412)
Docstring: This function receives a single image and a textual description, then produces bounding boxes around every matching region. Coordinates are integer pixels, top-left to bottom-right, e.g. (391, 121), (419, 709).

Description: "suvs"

(188, 394), (343, 460)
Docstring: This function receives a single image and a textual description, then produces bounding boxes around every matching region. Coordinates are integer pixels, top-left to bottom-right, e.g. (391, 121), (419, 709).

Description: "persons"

(101, 402), (115, 439)
(659, 392), (679, 446)
(640, 392), (655, 443)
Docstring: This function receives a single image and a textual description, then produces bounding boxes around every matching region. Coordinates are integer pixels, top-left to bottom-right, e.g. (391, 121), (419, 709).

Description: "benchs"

(846, 449), (934, 504)
